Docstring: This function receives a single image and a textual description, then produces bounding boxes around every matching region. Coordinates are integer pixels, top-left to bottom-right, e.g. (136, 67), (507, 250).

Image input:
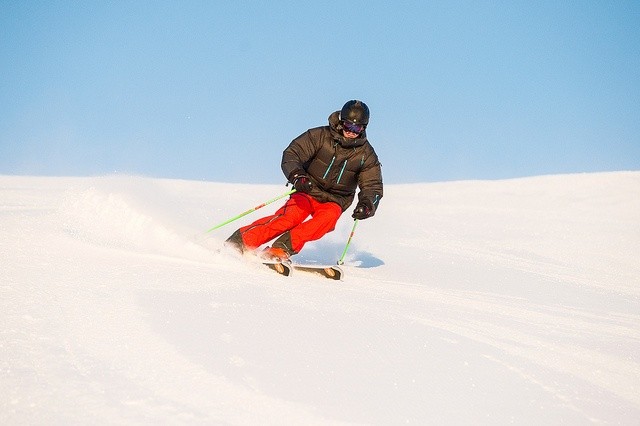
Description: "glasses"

(343, 121), (363, 133)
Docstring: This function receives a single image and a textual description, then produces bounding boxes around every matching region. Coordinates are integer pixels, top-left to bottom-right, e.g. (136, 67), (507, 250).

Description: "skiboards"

(260, 261), (344, 281)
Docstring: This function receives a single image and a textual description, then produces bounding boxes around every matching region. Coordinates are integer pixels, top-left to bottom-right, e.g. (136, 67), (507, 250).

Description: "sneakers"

(261, 247), (290, 264)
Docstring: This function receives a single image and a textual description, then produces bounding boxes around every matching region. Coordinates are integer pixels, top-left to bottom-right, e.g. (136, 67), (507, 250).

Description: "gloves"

(352, 203), (370, 219)
(294, 176), (312, 194)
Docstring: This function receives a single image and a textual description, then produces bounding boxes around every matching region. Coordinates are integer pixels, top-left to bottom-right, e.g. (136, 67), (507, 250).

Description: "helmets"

(339, 100), (370, 124)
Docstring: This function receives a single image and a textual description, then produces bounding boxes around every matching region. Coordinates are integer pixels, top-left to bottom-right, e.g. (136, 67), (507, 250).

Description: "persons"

(224, 100), (383, 264)
